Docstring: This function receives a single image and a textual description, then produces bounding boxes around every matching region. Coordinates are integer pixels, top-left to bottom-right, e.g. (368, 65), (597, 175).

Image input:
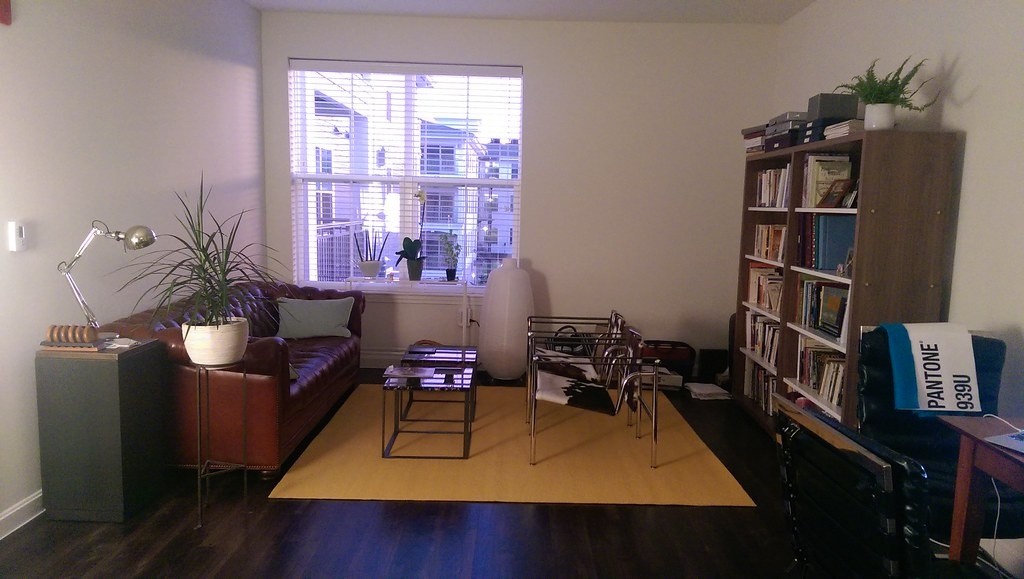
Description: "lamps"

(55, 219), (157, 343)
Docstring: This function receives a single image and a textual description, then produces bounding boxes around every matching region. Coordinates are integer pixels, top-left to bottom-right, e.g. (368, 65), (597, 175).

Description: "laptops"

(984, 431), (1024, 454)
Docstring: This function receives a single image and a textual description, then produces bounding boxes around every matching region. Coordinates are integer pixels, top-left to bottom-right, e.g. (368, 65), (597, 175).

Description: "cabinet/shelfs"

(732, 128), (966, 444)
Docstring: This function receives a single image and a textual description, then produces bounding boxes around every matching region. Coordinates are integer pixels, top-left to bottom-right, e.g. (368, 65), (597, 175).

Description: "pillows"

(275, 295), (354, 339)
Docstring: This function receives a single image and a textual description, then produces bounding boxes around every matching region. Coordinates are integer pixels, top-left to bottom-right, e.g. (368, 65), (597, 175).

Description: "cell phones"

(408, 347), (436, 354)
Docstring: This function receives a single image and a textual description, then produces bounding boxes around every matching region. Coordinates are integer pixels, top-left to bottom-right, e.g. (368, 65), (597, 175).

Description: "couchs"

(96, 282), (366, 482)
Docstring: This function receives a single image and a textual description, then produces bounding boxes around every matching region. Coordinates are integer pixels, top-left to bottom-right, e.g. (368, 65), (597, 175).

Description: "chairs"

(771, 392), (996, 579)
(526, 309), (662, 470)
(859, 323), (1007, 536)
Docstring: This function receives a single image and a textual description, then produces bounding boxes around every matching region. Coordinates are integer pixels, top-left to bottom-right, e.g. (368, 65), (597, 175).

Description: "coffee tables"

(382, 368), (475, 460)
(399, 345), (480, 423)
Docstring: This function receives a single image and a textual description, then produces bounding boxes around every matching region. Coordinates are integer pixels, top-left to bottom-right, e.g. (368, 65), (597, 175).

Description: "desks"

(938, 416), (1024, 567)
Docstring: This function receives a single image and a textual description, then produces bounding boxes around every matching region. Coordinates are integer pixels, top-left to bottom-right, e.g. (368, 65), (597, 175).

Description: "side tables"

(35, 338), (171, 524)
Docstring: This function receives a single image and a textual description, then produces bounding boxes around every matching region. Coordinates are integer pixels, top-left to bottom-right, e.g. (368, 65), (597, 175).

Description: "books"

(796, 213), (857, 272)
(747, 260), (784, 316)
(794, 334), (846, 407)
(753, 223), (787, 263)
(822, 119), (865, 141)
(756, 152), (853, 208)
(742, 356), (777, 417)
(381, 365), (436, 380)
(795, 275), (849, 339)
(743, 310), (781, 369)
(408, 346), (437, 354)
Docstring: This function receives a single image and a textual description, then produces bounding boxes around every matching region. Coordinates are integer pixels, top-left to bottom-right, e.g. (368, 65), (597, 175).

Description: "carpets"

(267, 384), (758, 508)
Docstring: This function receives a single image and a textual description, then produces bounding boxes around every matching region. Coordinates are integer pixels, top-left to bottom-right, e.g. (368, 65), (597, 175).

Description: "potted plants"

(834, 55), (941, 130)
(394, 237), (427, 281)
(112, 170), (296, 370)
(440, 229), (462, 282)
(352, 229), (390, 278)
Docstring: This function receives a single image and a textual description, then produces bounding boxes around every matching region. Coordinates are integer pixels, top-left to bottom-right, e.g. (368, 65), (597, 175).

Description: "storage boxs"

(740, 93), (858, 153)
